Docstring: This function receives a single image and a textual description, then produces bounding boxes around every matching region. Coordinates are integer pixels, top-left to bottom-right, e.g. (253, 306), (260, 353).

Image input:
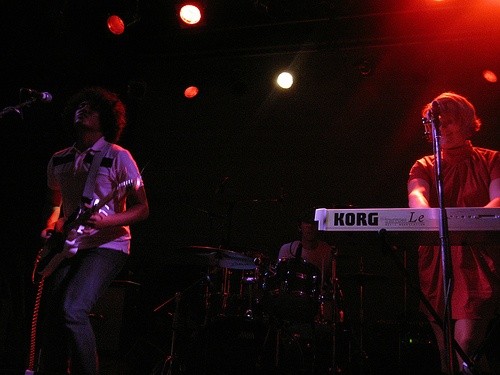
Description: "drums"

(221, 262), (260, 314)
(270, 258), (322, 319)
(195, 316), (315, 375)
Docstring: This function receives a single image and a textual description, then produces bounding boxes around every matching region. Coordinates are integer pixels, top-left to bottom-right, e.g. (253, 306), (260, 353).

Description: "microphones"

(19, 88), (52, 103)
(431, 100), (441, 127)
(295, 243), (302, 260)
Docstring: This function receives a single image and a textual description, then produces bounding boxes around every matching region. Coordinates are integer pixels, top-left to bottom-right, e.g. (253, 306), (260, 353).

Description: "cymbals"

(179, 246), (237, 258)
(351, 273), (376, 277)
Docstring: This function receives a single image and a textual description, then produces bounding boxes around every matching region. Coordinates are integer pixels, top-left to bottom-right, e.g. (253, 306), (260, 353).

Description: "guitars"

(39, 177), (142, 271)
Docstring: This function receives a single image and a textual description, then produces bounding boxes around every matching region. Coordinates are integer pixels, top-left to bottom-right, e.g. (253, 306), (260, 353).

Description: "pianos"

(314, 206), (500, 232)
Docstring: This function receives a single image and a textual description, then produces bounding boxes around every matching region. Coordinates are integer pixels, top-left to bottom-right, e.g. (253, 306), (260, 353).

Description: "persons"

(22, 89), (149, 375)
(408, 92), (500, 375)
(277, 209), (334, 275)
